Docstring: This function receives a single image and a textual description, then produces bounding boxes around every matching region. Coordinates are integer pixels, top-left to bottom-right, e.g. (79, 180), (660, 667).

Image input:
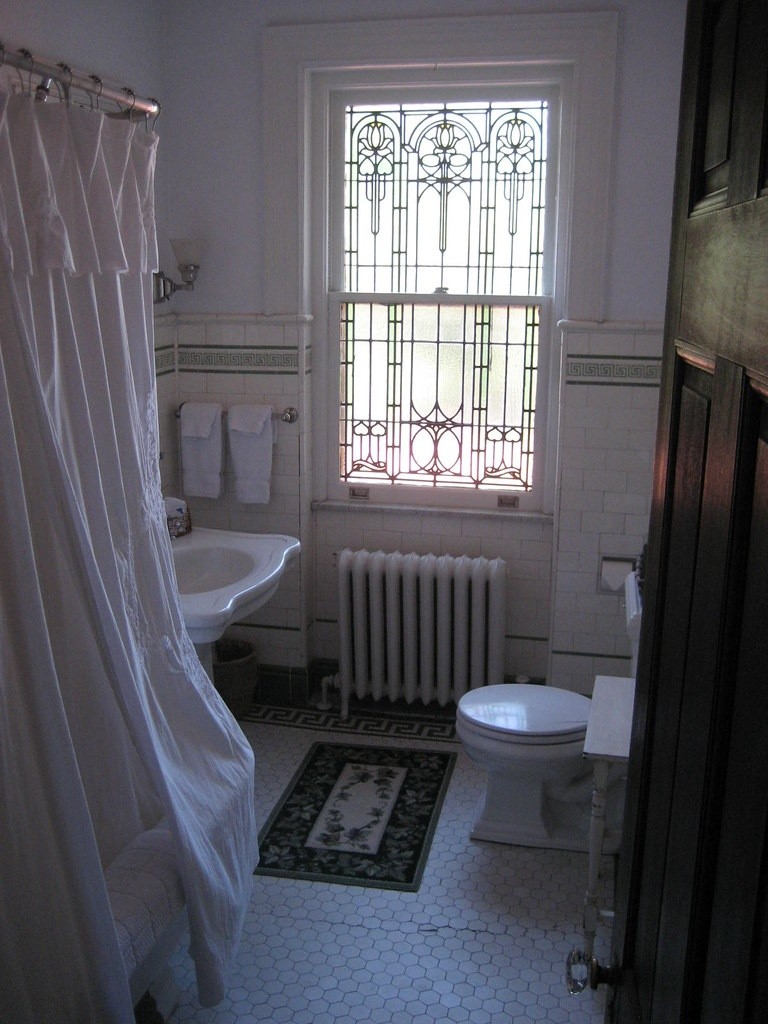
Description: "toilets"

(456, 571), (641, 854)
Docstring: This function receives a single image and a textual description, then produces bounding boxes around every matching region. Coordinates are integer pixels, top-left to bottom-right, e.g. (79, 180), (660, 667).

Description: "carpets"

(252, 741), (458, 893)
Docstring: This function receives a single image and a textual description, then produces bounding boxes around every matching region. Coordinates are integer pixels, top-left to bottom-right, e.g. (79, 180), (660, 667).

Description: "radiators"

(317, 545), (507, 722)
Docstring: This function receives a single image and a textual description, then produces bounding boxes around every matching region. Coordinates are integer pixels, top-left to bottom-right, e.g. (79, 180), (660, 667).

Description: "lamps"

(153, 237), (200, 305)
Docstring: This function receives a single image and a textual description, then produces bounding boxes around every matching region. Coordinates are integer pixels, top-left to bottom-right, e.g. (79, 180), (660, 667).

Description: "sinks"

(170, 527), (302, 646)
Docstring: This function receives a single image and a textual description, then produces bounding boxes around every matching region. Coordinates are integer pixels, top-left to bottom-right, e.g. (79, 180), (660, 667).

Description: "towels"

(180, 402), (223, 500)
(228, 404), (280, 505)
